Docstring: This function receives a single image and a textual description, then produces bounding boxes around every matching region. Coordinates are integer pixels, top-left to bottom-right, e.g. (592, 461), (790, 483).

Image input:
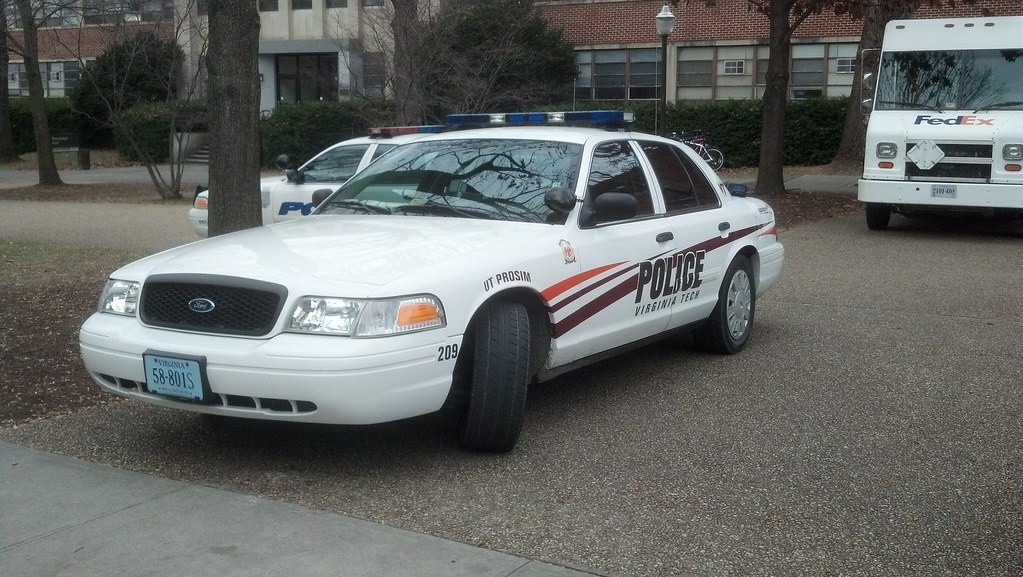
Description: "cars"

(189, 133), (443, 238)
(79, 125), (786, 454)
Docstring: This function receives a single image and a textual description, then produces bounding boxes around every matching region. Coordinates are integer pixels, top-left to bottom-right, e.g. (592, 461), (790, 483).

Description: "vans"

(858, 16), (1023, 230)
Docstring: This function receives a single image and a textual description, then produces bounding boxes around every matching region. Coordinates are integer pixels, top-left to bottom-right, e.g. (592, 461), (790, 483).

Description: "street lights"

(656, 5), (675, 136)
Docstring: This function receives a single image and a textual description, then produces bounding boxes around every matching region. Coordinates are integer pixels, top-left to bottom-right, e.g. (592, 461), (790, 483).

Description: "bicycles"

(669, 130), (724, 173)
